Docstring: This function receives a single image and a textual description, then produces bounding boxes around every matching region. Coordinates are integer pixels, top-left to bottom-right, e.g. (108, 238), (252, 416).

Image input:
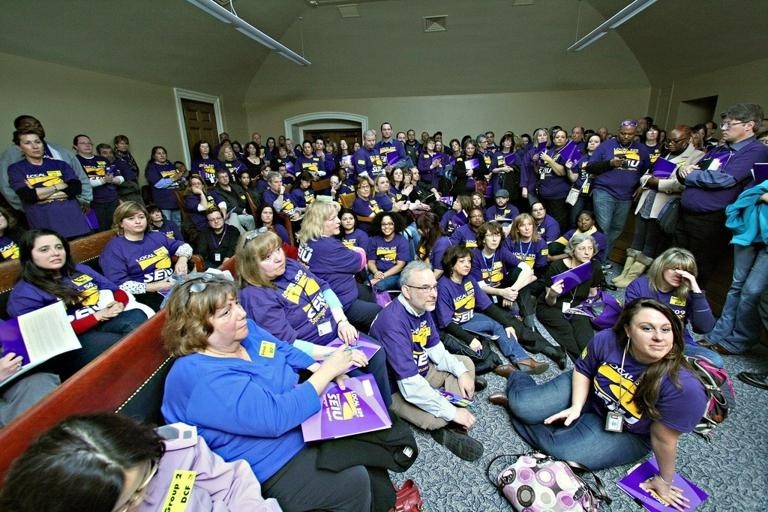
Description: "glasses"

(405, 282), (439, 292)
(183, 272), (218, 310)
(243, 226), (269, 251)
(618, 120), (639, 127)
(719, 121), (746, 130)
(118, 460), (160, 512)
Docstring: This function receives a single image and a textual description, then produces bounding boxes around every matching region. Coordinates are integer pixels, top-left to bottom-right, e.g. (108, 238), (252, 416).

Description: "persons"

(0, 105), (767, 511)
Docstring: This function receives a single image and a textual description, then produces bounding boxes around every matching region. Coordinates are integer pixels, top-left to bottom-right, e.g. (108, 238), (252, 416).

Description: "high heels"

(517, 357), (550, 375)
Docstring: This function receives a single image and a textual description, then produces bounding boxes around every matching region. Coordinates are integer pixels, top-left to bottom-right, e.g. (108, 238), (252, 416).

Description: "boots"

(610, 247), (643, 283)
(613, 252), (655, 290)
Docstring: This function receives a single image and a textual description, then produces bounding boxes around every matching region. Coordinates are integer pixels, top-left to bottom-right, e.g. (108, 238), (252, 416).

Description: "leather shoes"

(737, 368), (768, 390)
(709, 343), (753, 355)
(487, 390), (508, 407)
(552, 344), (568, 371)
(522, 316), (542, 336)
(696, 338), (714, 347)
(494, 363), (517, 380)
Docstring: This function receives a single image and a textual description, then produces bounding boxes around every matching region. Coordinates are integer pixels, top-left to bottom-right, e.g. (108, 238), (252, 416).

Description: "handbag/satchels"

(441, 326), (503, 374)
(658, 196), (685, 238)
(565, 289), (622, 331)
(486, 446), (613, 512)
(678, 354), (739, 442)
(387, 478), (424, 512)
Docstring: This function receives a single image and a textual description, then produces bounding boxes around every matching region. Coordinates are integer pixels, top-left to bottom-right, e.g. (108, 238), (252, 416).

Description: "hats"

(495, 188), (509, 198)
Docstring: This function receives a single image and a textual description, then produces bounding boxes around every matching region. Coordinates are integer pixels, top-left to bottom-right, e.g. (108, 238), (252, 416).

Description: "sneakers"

(475, 374), (487, 392)
(430, 420), (484, 462)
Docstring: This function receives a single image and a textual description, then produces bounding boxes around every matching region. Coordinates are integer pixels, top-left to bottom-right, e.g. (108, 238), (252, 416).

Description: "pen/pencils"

(323, 344), (364, 357)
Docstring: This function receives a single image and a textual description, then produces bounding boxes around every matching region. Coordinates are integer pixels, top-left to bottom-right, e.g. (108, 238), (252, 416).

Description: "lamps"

(567, 0), (659, 53)
(186, 0), (312, 68)
